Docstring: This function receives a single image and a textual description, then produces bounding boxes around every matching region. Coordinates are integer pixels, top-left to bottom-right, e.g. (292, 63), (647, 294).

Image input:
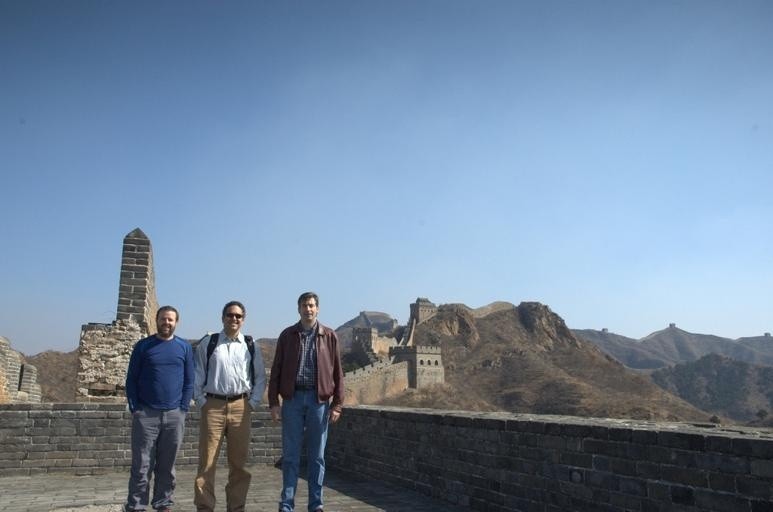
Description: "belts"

(207, 392), (246, 401)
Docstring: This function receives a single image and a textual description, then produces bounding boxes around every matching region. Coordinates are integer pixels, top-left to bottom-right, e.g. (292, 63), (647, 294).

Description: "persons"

(267, 291), (345, 512)
(123, 304), (193, 511)
(190, 300), (268, 512)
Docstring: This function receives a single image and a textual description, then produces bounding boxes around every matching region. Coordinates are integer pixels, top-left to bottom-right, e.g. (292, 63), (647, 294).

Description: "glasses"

(226, 314), (242, 318)
